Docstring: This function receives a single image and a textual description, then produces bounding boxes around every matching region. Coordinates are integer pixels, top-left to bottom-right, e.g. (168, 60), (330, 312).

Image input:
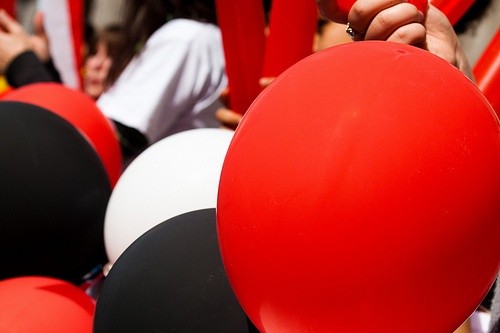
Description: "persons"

(216, 1), (500, 131)
(1, 1), (243, 173)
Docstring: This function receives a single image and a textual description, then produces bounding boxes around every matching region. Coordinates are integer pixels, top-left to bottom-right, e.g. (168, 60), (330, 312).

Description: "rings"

(345, 22), (367, 41)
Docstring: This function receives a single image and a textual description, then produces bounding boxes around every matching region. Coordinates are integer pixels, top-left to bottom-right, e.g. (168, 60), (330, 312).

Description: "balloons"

(92, 207), (261, 333)
(0, 83), (122, 293)
(103, 128), (236, 277)
(0, 276), (96, 333)
(216, 40), (500, 333)
